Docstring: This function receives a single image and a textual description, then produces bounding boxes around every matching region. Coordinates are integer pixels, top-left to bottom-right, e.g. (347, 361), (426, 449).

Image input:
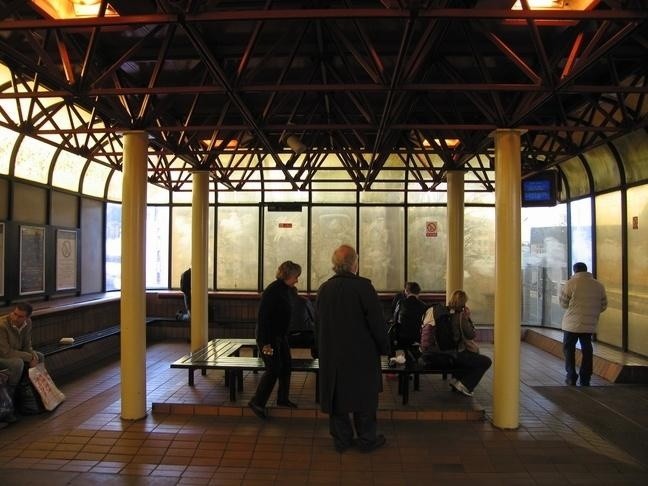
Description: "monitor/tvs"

(522, 170), (559, 207)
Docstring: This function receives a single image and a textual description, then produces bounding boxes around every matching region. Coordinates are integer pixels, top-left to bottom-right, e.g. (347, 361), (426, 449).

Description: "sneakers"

(277, 401), (295, 408)
(565, 379), (576, 385)
(453, 381), (474, 397)
(338, 438), (357, 451)
(248, 403), (267, 421)
(449, 379), (457, 386)
(359, 435), (384, 452)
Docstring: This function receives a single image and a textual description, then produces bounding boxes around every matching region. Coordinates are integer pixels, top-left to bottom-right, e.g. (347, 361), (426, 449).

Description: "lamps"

(286, 134), (307, 155)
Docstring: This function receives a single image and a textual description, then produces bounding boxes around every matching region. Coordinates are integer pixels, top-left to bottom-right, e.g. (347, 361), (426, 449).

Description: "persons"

(385, 281), (429, 357)
(311, 245), (397, 455)
(391, 282), (407, 314)
(0, 301), (45, 422)
(248, 260), (302, 419)
(559, 262), (607, 386)
(441, 289), (492, 397)
(175, 267), (191, 344)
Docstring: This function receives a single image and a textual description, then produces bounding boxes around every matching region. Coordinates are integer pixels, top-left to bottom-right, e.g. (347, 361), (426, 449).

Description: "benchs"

(239, 336), (259, 347)
(407, 341), (450, 392)
(168, 355), (451, 407)
(140, 314), (257, 327)
(169, 335), (243, 385)
(1, 315), (151, 377)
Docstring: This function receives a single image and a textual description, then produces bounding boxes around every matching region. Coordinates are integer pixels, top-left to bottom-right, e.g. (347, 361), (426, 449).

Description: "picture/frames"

(0, 222), (5, 296)
(18, 224), (47, 296)
(55, 228), (78, 292)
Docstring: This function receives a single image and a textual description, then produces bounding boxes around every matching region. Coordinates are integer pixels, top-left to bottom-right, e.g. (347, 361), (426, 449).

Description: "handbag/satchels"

(417, 347), (460, 370)
(17, 381), (45, 416)
(431, 304), (461, 351)
(459, 338), (478, 353)
